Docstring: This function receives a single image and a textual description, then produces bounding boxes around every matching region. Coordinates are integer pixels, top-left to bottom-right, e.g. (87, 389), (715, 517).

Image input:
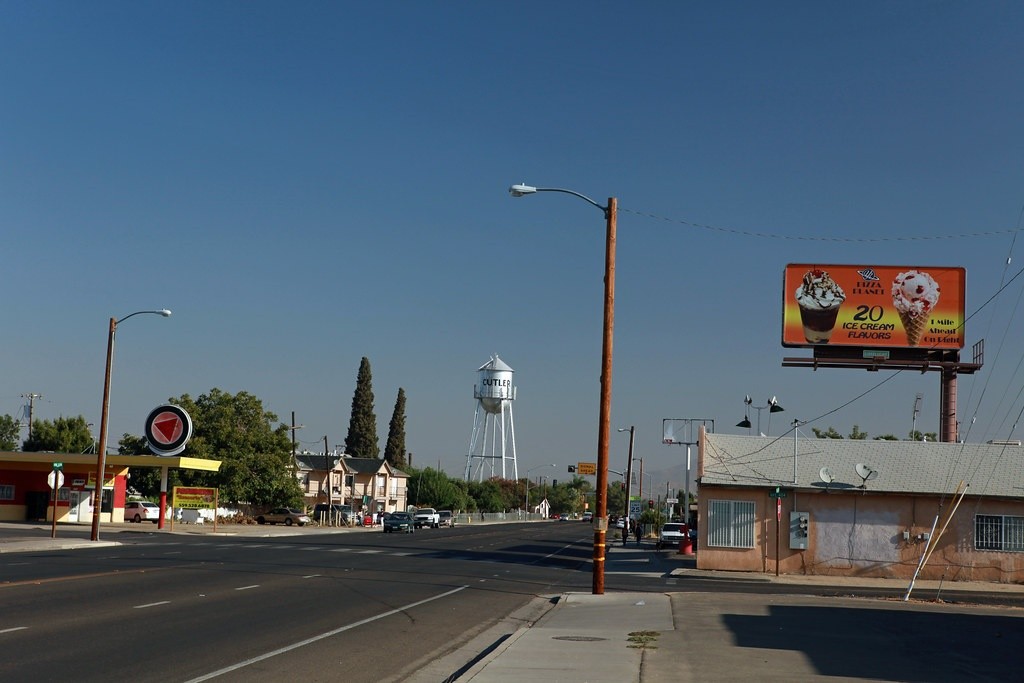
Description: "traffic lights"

(622, 483), (625, 491)
(649, 500), (653, 508)
(568, 465), (575, 472)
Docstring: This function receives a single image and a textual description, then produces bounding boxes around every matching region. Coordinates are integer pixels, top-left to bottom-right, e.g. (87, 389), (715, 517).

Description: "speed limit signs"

(630, 500), (641, 514)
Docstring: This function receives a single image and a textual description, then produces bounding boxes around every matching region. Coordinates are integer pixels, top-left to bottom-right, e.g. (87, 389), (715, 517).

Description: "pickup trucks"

(414, 508), (440, 530)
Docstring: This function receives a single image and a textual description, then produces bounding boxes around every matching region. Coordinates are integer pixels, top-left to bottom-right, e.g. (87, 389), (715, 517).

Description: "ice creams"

(795, 270), (846, 344)
(891, 270), (940, 346)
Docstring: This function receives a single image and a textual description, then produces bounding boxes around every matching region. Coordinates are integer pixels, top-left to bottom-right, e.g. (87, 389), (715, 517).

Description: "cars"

(363, 515), (372, 525)
(124, 501), (160, 524)
(608, 515), (618, 526)
(437, 510), (454, 528)
(254, 507), (311, 526)
(384, 512), (414, 534)
(551, 513), (560, 519)
(559, 513), (569, 521)
(660, 522), (692, 548)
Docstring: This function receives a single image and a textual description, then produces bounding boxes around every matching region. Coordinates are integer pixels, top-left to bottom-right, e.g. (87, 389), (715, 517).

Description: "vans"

(314, 503), (359, 526)
(582, 511), (592, 522)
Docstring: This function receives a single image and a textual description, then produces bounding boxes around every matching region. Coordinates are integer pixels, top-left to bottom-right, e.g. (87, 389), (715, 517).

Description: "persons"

(622, 526), (628, 547)
(379, 509), (384, 526)
(590, 514), (593, 523)
(481, 512), (485, 521)
(629, 519), (643, 545)
(357, 510), (363, 526)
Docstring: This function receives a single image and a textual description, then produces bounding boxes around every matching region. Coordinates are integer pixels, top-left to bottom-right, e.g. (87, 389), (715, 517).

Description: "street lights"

(525, 463), (557, 521)
(624, 468), (653, 536)
(507, 182), (619, 596)
(91, 310), (174, 542)
(617, 425), (634, 534)
